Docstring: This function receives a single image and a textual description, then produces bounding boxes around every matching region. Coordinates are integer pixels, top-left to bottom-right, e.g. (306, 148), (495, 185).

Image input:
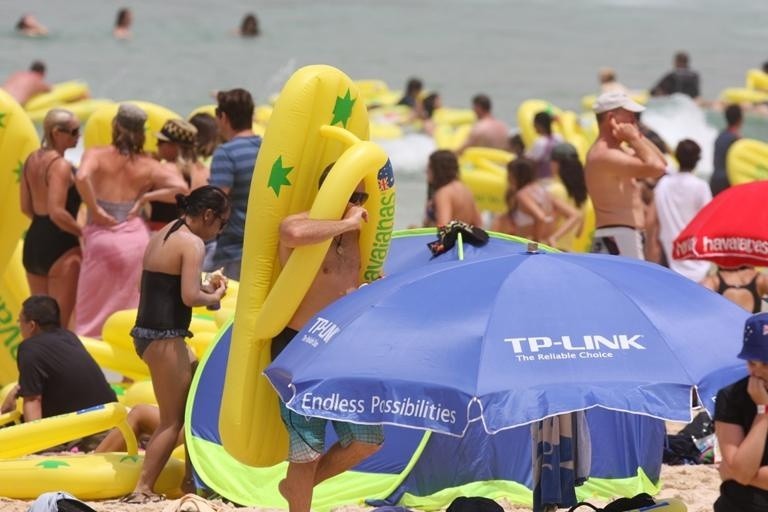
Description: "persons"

(113, 10), (133, 39)
(17, 15), (48, 37)
(229, 14), (263, 39)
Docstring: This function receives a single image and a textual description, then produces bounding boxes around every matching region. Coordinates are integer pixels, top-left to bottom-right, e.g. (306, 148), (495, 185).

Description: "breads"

(201, 270), (228, 294)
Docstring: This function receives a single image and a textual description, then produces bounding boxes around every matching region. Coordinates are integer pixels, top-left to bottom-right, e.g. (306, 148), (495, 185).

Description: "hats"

(589, 92), (646, 116)
(737, 313), (768, 364)
(151, 118), (204, 150)
(115, 101), (149, 134)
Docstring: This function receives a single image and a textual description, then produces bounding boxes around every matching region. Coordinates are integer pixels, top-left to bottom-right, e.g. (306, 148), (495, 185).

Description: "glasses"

(58, 125), (80, 138)
(349, 191), (369, 207)
(216, 213), (230, 230)
